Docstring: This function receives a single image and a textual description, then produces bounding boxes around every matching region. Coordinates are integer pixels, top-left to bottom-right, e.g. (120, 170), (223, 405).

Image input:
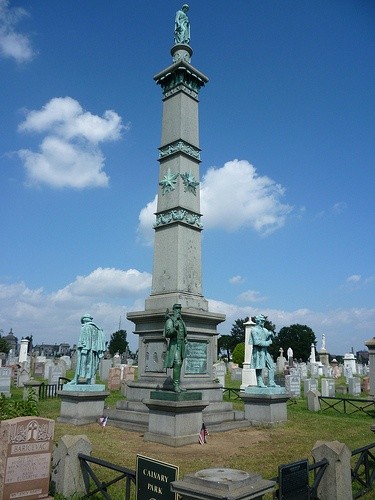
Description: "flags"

(95, 416), (107, 426)
(198, 423), (209, 445)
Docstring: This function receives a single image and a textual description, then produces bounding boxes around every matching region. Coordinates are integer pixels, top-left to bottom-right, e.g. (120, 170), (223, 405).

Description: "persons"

(162, 304), (187, 392)
(248, 314), (281, 388)
(67, 313), (107, 385)
(173, 4), (190, 45)
